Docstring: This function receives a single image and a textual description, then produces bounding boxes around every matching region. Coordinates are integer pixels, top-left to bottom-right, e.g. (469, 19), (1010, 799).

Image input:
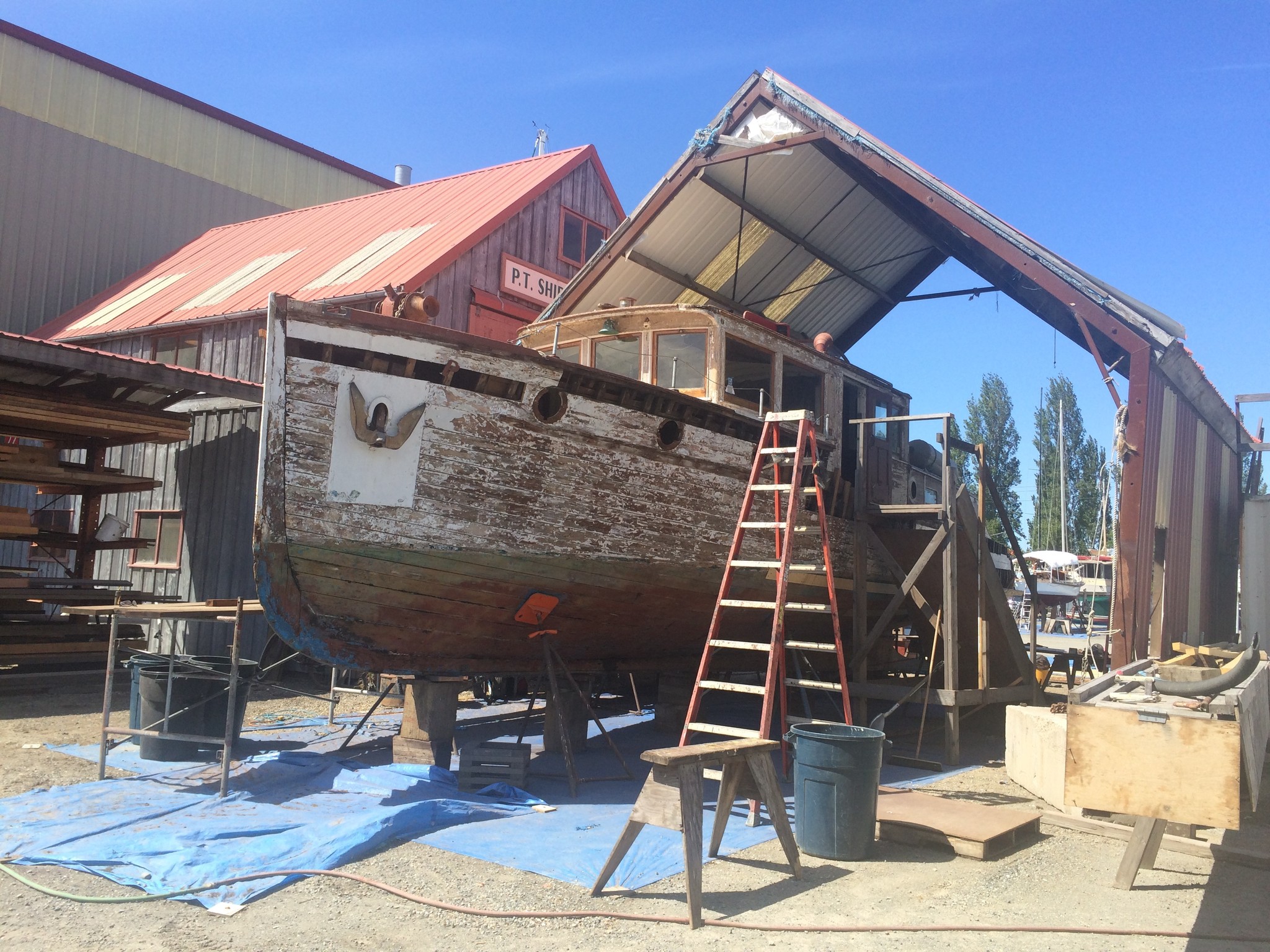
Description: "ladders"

(680, 411), (854, 828)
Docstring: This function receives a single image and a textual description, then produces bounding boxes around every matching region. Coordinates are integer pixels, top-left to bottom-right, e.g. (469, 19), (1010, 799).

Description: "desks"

(58, 598), (264, 801)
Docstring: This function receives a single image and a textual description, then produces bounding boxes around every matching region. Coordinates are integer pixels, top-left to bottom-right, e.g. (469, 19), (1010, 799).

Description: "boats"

(247, 284), (956, 674)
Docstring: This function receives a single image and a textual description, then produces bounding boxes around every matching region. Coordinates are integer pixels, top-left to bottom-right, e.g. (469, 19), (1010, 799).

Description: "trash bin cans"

(123, 653), (259, 761)
(779, 723), (893, 858)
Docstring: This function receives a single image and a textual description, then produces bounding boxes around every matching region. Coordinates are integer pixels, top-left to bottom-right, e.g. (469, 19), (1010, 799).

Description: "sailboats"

(1011, 388), (1113, 623)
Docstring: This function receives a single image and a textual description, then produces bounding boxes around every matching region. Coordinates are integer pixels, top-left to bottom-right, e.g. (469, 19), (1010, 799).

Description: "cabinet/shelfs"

(0, 394), (193, 673)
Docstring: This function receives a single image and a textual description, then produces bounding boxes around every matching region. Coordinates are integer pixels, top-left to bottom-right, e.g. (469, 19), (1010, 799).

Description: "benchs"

(590, 728), (805, 927)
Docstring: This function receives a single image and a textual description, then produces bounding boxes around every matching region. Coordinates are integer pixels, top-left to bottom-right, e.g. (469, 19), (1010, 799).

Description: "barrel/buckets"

(138, 664), (213, 763)
(187, 656), (262, 750)
(124, 653), (194, 745)
(96, 513), (130, 542)
(784, 724), (892, 861)
